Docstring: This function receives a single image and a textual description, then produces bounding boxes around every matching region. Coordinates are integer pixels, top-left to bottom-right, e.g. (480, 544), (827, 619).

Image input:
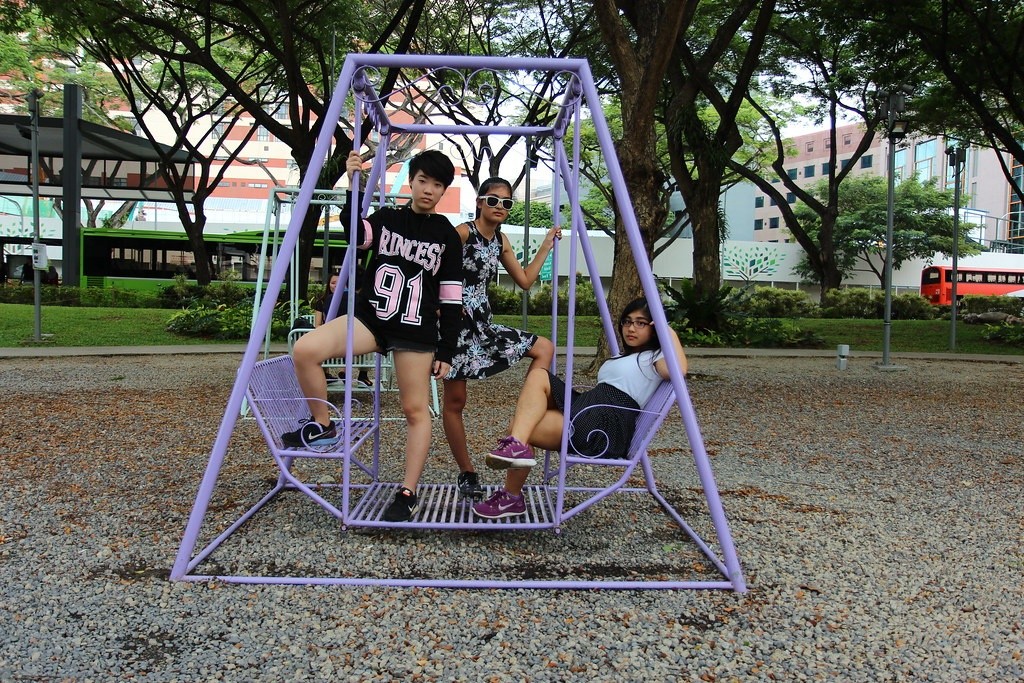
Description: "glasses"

(479, 196), (514, 210)
(621, 319), (650, 329)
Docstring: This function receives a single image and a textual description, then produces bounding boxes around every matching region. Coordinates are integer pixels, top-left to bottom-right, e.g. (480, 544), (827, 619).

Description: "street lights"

(24, 90), (45, 340)
(882, 92), (906, 364)
(523, 153), (538, 333)
(468, 213), (474, 221)
(944, 146), (965, 349)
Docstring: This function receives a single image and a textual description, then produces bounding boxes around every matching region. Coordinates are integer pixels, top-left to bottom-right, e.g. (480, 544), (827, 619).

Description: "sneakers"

(471, 487), (527, 518)
(458, 471), (483, 495)
(485, 436), (539, 470)
(281, 416), (337, 446)
(385, 487), (417, 521)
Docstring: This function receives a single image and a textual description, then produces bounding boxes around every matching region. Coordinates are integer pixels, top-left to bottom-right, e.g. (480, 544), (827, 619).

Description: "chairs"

(237, 314), (439, 533)
(543, 374), (680, 535)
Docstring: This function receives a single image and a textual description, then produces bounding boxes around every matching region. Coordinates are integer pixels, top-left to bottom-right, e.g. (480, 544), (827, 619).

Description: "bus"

(920, 267), (1024, 307)
(79, 225), (373, 306)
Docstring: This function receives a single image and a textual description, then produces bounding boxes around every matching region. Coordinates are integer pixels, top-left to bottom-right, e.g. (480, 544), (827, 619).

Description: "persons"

(431, 175), (562, 497)
(0, 256), (10, 285)
(282, 151), (463, 524)
(18, 256), (58, 288)
(471, 295), (688, 519)
